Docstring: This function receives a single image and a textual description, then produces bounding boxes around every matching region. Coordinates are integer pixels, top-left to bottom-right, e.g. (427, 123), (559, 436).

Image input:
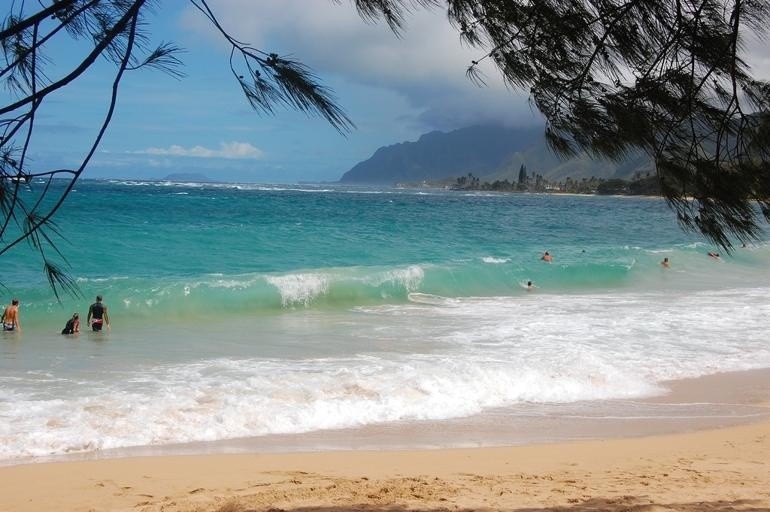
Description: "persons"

(539, 250), (554, 262)
(1, 298), (22, 335)
(741, 242), (747, 248)
(526, 280), (535, 290)
(85, 293), (112, 334)
(581, 249), (586, 253)
(659, 256), (670, 269)
(709, 251), (721, 261)
(60, 312), (81, 336)
